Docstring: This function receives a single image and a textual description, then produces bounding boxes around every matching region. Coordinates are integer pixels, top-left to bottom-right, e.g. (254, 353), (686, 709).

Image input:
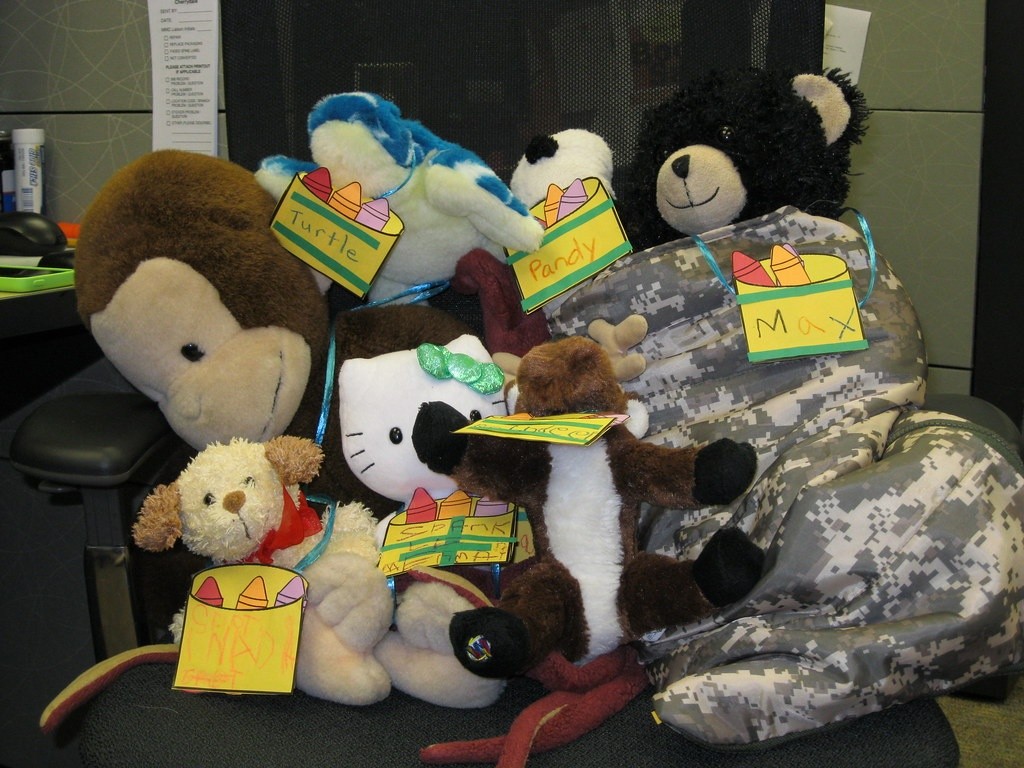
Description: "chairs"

(9, 0), (1024, 768)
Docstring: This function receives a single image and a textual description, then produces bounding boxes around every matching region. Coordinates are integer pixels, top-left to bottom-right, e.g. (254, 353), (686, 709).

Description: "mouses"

(0, 211), (68, 257)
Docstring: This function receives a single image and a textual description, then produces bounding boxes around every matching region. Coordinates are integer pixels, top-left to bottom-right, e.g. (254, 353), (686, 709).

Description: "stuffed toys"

(74, 68), (871, 768)
